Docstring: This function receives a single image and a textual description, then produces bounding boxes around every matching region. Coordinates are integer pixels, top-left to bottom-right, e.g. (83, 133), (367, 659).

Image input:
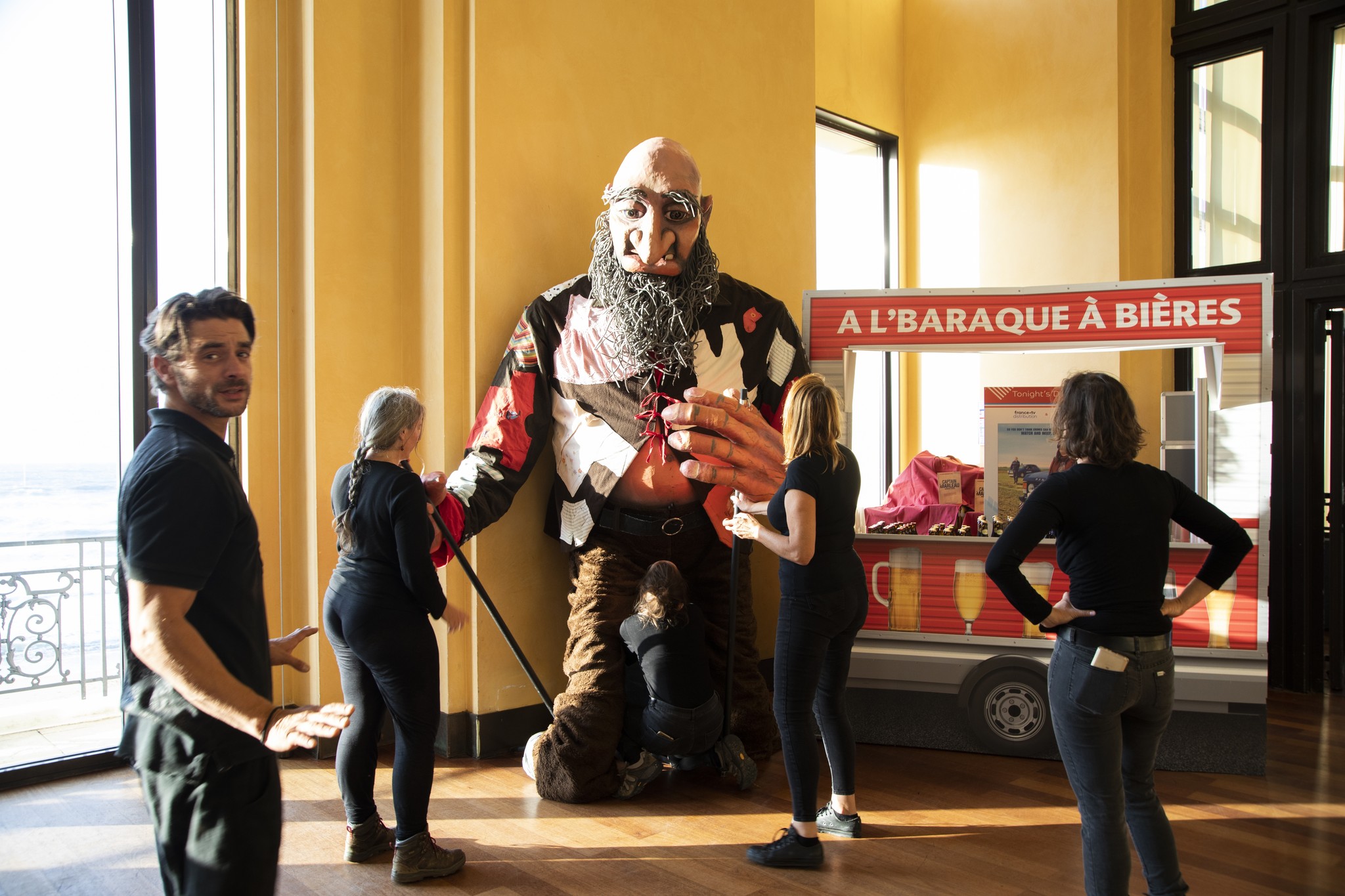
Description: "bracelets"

(260, 705), (289, 746)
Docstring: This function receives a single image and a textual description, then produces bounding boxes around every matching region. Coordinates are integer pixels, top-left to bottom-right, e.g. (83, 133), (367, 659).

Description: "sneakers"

(344, 812), (397, 863)
(715, 734), (758, 791)
(389, 823), (466, 885)
(816, 801), (862, 839)
(746, 824), (824, 866)
(614, 747), (663, 800)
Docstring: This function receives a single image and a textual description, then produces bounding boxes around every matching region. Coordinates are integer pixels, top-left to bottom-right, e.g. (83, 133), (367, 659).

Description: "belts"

(1038, 622), (1173, 653)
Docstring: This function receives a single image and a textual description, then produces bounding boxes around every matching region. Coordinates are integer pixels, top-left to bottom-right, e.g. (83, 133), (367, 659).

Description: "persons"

(322, 384), (470, 882)
(1047, 441), (1077, 476)
(982, 369), (1254, 895)
(1026, 466), (1030, 470)
(723, 371), (870, 868)
(414, 134), (817, 805)
(119, 285), (354, 896)
(1010, 457), (1020, 485)
(619, 558), (752, 798)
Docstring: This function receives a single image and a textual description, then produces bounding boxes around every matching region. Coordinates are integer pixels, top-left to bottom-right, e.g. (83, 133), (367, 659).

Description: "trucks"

(801, 279), (1270, 761)
